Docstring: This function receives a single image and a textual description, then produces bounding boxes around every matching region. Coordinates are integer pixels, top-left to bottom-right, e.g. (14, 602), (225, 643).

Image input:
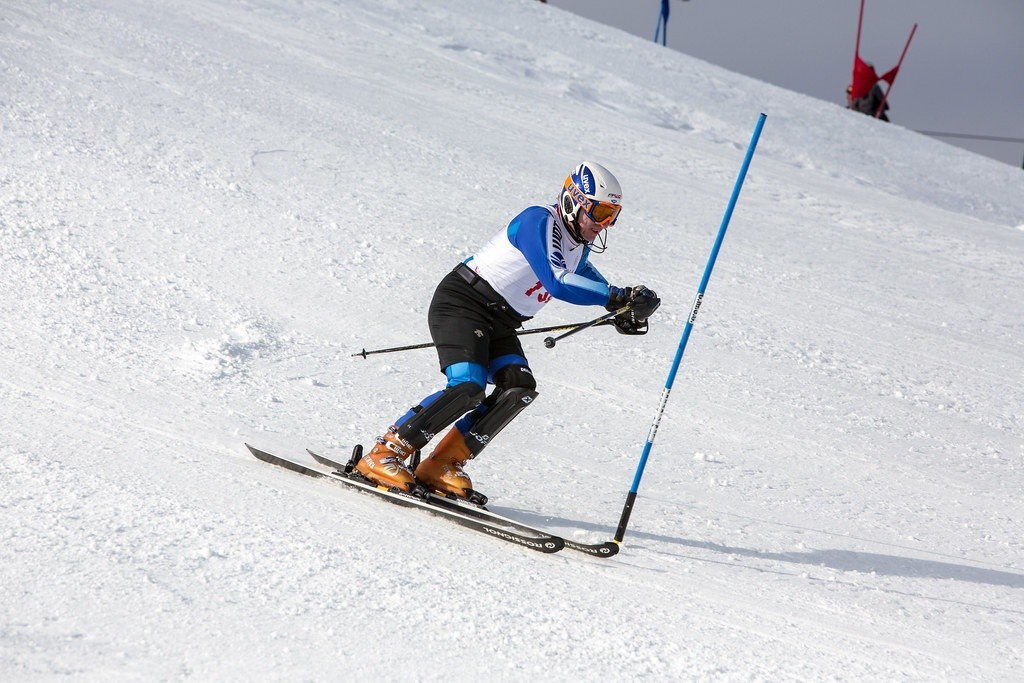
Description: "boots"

(355, 425), (416, 492)
(414, 425), (474, 498)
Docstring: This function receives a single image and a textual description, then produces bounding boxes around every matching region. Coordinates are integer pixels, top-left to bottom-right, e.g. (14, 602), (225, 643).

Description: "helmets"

(558, 161), (622, 222)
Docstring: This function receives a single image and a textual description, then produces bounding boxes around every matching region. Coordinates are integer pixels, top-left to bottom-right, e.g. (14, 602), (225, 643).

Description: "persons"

(845, 64), (891, 124)
(358, 159), (662, 501)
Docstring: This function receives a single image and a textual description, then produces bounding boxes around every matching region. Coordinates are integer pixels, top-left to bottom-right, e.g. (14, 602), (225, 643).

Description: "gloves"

(604, 306), (648, 332)
(605, 284), (657, 317)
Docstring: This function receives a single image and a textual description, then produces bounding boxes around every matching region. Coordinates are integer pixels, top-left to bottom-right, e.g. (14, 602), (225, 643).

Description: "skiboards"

(244, 443), (621, 560)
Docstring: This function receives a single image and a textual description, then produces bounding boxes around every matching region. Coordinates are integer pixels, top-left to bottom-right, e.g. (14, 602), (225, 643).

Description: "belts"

(453, 262), (501, 303)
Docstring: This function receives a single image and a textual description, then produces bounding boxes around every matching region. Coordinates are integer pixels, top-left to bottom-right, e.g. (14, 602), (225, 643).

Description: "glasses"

(564, 172), (623, 227)
